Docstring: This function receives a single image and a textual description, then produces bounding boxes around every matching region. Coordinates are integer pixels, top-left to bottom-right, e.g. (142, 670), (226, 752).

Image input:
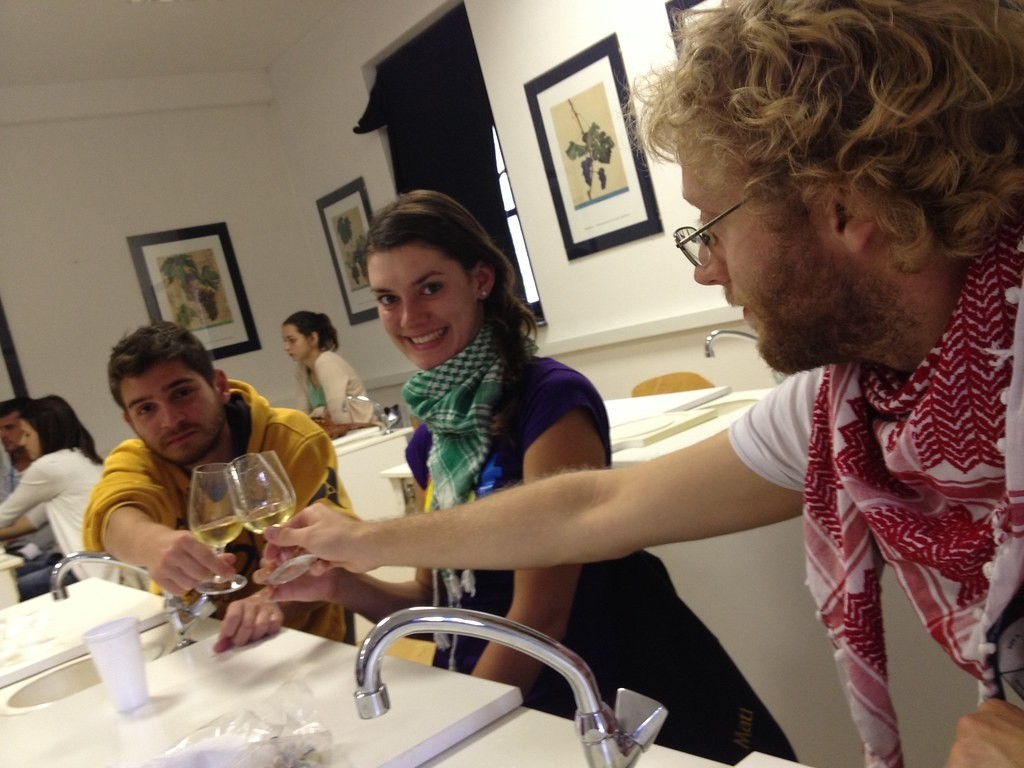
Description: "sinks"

(7, 640), (165, 717)
(698, 398), (761, 418)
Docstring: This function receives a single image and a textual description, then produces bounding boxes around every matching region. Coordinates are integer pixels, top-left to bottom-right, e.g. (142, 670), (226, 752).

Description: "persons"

(83, 322), (347, 652)
(0, 395), (104, 601)
(241, 190), (797, 765)
(281, 311), (370, 436)
(263, 0), (1023, 768)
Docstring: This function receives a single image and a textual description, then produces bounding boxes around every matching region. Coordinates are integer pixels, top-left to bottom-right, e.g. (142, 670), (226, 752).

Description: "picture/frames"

(523, 32), (664, 262)
(127, 221), (263, 363)
(314, 176), (380, 329)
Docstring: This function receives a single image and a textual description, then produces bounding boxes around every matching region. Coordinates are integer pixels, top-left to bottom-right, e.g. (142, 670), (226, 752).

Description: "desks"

(0, 386), (1024, 768)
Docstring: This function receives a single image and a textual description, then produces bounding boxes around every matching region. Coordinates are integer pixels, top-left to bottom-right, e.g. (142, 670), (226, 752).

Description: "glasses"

(674, 180), (780, 269)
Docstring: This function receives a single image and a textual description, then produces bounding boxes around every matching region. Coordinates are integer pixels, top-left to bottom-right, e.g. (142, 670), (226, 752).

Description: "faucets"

(352, 603), (672, 768)
(48, 549), (219, 650)
(704, 328), (759, 358)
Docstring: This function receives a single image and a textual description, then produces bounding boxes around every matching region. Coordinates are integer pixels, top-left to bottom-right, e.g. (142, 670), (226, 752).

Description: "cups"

(83, 619), (149, 711)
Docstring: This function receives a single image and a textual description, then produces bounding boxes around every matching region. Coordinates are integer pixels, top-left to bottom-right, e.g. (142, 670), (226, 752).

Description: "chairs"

(631, 373), (713, 399)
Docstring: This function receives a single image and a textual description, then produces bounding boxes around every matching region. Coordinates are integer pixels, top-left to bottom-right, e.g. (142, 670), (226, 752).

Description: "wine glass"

(186, 463), (248, 594)
(225, 450), (318, 586)
